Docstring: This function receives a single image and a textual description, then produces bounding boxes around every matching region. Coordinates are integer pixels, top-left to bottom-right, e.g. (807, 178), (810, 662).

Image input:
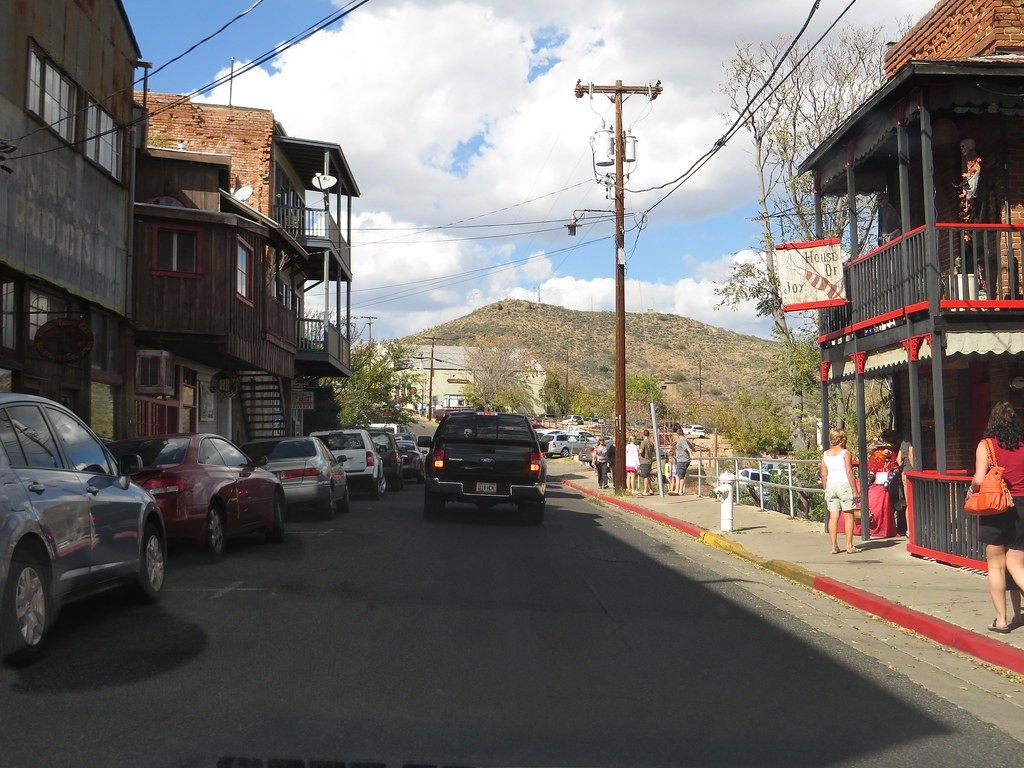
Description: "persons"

(667, 428), (695, 496)
(591, 436), (609, 489)
(625, 435), (641, 493)
(639, 430), (654, 495)
(896, 420), (913, 536)
(607, 437), (615, 489)
(966, 402), (1024, 633)
(820, 429), (862, 553)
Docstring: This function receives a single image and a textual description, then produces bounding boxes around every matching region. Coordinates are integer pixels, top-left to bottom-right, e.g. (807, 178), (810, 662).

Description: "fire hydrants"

(714, 470), (737, 532)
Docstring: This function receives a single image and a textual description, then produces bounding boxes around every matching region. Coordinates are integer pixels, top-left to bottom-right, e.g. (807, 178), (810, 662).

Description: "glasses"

(600, 439), (604, 441)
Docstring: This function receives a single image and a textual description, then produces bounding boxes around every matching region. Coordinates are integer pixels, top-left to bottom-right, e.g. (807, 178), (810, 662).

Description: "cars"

(0, 393), (167, 667)
(533, 428), (562, 441)
(578, 442), (666, 469)
(393, 433), (428, 482)
(103, 433), (289, 564)
(239, 436), (349, 520)
(732, 468), (772, 495)
(762, 462), (801, 481)
(368, 429), (402, 488)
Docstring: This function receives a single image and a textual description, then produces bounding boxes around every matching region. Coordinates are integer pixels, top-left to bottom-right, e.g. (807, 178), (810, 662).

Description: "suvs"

(418, 411), (549, 522)
(682, 424), (705, 435)
(309, 427), (386, 499)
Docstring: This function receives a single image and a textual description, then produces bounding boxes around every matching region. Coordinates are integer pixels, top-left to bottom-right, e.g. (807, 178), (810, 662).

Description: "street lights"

(564, 209), (626, 496)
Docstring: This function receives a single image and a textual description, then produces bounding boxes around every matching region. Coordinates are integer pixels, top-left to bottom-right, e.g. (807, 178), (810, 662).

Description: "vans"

(541, 432), (590, 458)
(564, 415), (584, 425)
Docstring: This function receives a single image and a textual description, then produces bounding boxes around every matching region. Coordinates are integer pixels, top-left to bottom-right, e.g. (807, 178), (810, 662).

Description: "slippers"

(988, 618), (1009, 633)
(638, 491), (650, 496)
(649, 490), (654, 495)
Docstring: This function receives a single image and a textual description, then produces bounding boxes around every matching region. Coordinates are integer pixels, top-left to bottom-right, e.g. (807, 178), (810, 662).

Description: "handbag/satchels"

(963, 438), (1015, 517)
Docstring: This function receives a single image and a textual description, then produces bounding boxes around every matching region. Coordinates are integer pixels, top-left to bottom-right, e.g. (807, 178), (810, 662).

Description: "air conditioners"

(136, 350), (174, 396)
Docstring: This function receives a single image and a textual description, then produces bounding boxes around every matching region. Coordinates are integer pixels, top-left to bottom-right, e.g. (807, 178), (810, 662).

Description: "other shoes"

(847, 546), (861, 554)
(632, 490), (639, 494)
(896, 532), (906, 537)
(832, 547), (840, 554)
(604, 485), (609, 489)
(667, 491), (683, 496)
(613, 486), (616, 489)
(598, 485), (602, 489)
